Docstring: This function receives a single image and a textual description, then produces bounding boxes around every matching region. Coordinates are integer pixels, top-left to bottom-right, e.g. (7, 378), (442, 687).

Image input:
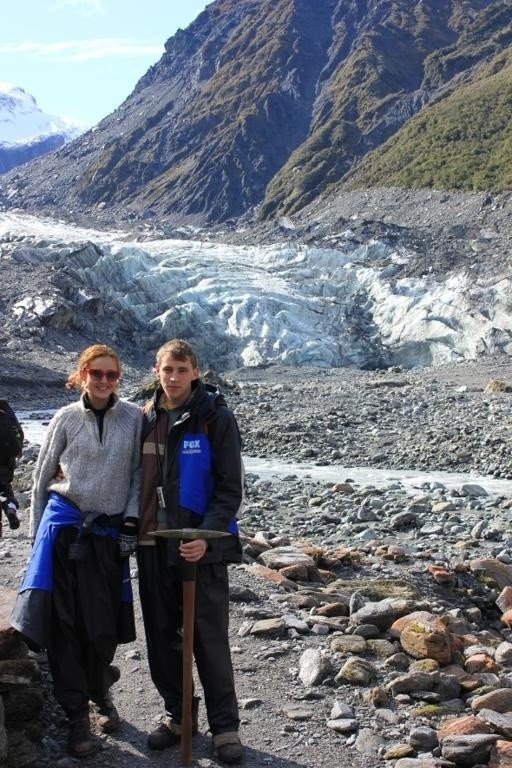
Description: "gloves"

(114, 524), (138, 568)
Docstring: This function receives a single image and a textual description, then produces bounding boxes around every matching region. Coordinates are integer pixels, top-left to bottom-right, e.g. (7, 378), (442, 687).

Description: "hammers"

(147, 528), (232, 768)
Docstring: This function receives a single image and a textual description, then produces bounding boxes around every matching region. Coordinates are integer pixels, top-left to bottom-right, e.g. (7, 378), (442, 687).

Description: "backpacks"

(2, 408), (24, 453)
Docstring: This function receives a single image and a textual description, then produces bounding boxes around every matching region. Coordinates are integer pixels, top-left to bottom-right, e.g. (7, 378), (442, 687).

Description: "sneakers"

(146, 717), (200, 750)
(67, 712), (93, 756)
(88, 696), (120, 733)
(211, 730), (244, 762)
(5, 507), (20, 530)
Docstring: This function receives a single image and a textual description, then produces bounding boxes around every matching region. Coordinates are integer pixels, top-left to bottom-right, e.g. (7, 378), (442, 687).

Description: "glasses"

(83, 367), (120, 383)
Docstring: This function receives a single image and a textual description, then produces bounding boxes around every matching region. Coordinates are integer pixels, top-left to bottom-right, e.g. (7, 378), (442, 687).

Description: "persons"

(27, 341), (143, 760)
(54, 336), (249, 763)
(0, 397), (27, 539)
